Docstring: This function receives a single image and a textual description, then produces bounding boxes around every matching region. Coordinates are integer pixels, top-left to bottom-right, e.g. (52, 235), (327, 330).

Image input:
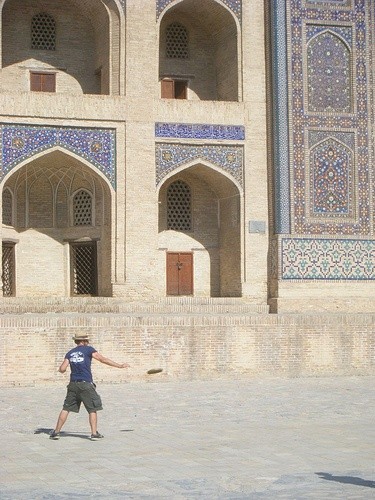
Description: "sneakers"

(50, 431), (60, 439)
(91, 431), (104, 441)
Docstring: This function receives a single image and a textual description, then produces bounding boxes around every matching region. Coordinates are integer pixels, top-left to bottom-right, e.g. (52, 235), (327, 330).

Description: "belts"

(70, 380), (88, 382)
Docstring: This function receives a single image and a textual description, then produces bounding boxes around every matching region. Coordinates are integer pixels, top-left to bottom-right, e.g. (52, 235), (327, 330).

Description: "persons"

(50, 331), (130, 441)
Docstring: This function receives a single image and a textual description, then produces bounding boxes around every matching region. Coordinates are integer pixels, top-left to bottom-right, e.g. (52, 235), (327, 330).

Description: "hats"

(72, 332), (89, 340)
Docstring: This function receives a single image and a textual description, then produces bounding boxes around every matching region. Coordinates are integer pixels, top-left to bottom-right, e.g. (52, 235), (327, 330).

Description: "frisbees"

(147, 368), (163, 374)
